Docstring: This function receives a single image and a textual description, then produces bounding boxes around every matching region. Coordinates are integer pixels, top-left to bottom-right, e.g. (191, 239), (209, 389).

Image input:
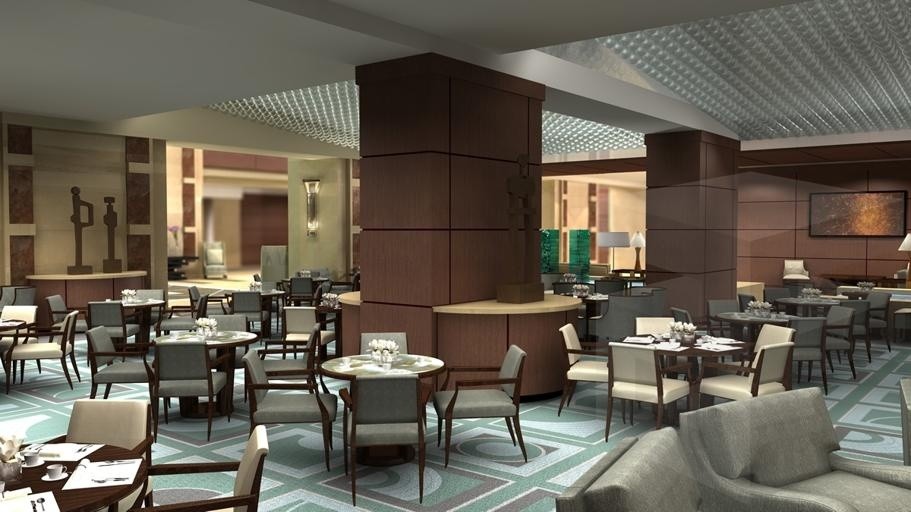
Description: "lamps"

(595, 230), (631, 272)
(303, 176), (322, 237)
(628, 232), (645, 272)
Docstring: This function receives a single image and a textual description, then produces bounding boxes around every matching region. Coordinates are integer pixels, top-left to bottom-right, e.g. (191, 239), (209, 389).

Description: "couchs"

(557, 425), (856, 509)
(680, 388), (910, 511)
(201, 242), (227, 278)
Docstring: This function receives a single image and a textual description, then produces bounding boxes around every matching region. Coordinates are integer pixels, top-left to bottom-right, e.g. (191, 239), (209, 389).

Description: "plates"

(23, 458), (48, 469)
(41, 472), (70, 482)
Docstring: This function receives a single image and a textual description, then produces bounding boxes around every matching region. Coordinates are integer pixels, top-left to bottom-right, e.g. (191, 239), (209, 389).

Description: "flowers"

(367, 338), (399, 355)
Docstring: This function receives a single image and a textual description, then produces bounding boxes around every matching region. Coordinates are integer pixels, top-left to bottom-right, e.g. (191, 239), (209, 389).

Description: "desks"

(617, 336), (749, 413)
(1, 441), (148, 511)
(152, 329), (259, 419)
(319, 353), (446, 468)
(1, 320), (26, 331)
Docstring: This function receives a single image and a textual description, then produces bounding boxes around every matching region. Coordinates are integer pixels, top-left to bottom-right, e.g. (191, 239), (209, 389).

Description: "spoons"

(38, 497), (46, 511)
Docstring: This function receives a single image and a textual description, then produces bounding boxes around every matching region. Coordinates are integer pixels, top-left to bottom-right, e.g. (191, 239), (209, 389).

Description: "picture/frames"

(807, 191), (908, 237)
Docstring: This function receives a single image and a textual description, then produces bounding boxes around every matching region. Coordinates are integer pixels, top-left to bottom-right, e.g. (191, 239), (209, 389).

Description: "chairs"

(556, 323), (625, 424)
(604, 341), (691, 445)
(5, 309), (80, 389)
(783, 260), (812, 290)
(1, 305), (42, 384)
(207, 315), (251, 403)
(634, 316), (691, 416)
(540, 272), (649, 316)
(435, 343), (527, 470)
(46, 289), (215, 364)
(705, 323), (797, 389)
(338, 373), (433, 506)
(86, 324), (171, 422)
(188, 272), (360, 346)
(669, 275), (911, 364)
(153, 342), (231, 441)
(137, 425), (272, 512)
(699, 341), (796, 408)
(44, 399), (155, 512)
(1, 285), (33, 312)
(358, 331), (408, 353)
(243, 322), (321, 401)
(784, 317), (829, 395)
(240, 348), (338, 472)
(798, 306), (858, 382)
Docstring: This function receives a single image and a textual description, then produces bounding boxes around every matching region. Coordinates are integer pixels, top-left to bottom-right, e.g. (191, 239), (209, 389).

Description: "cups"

(23, 452), (40, 464)
(342, 357), (351, 364)
(384, 364), (391, 370)
(417, 357), (426, 363)
(47, 463), (68, 478)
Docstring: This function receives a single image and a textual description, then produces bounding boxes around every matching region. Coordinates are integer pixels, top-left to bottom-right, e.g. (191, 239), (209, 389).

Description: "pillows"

(585, 425), (703, 511)
(206, 249), (224, 264)
(697, 387), (843, 487)
